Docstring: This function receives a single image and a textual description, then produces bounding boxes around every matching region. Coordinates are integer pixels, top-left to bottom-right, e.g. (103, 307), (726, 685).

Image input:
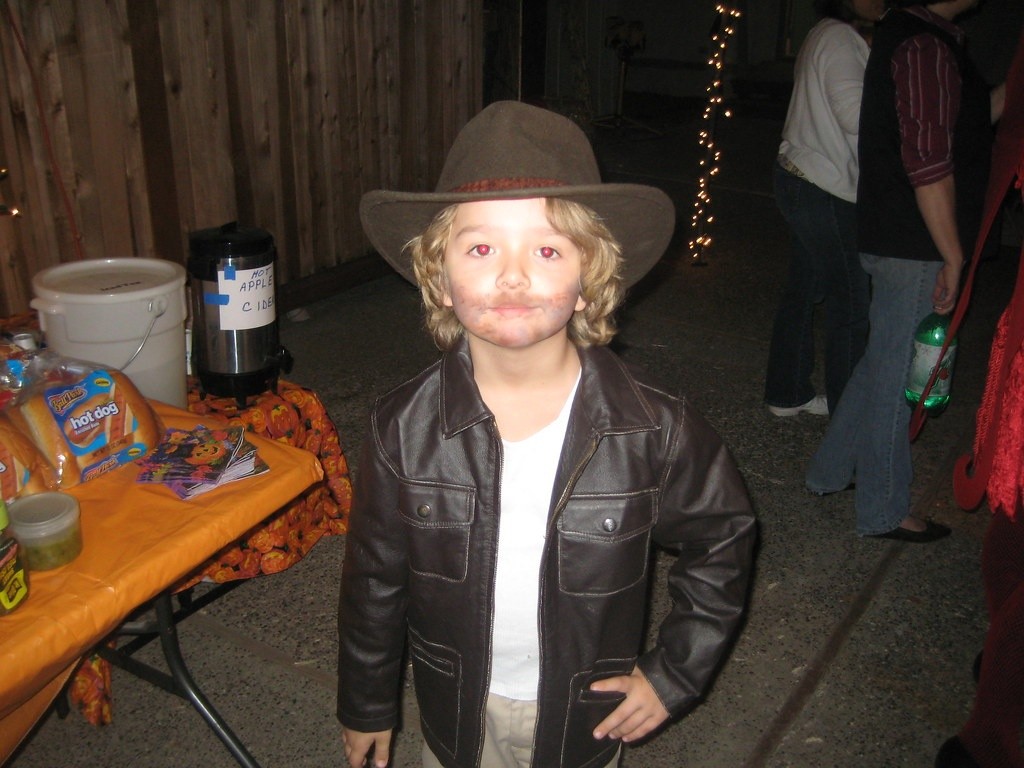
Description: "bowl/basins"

(6, 492), (83, 571)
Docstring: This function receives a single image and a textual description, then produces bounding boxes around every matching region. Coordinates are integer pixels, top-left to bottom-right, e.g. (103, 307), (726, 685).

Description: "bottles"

(905, 299), (957, 415)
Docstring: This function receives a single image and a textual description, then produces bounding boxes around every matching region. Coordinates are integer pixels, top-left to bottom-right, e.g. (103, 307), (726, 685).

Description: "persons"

(767, 0), (1024, 543)
(334, 99), (760, 768)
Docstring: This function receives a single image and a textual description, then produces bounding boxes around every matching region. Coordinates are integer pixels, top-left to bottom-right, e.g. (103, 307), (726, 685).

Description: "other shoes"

(804, 470), (857, 497)
(862, 515), (953, 544)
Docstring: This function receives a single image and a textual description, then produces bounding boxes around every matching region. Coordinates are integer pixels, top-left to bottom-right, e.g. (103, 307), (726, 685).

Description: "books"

(134, 426), (271, 499)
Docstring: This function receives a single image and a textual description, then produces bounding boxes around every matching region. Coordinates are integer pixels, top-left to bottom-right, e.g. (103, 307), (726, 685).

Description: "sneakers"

(768, 395), (830, 417)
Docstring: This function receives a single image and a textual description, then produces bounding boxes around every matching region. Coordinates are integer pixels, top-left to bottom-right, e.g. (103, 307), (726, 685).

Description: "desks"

(0, 398), (325, 768)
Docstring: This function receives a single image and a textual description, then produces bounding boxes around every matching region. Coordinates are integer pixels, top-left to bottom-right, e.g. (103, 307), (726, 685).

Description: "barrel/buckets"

(187, 222), (295, 409)
(29, 257), (188, 411)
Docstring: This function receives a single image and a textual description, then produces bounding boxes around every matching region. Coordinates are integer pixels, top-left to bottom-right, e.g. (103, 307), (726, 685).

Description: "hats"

(360, 100), (676, 292)
